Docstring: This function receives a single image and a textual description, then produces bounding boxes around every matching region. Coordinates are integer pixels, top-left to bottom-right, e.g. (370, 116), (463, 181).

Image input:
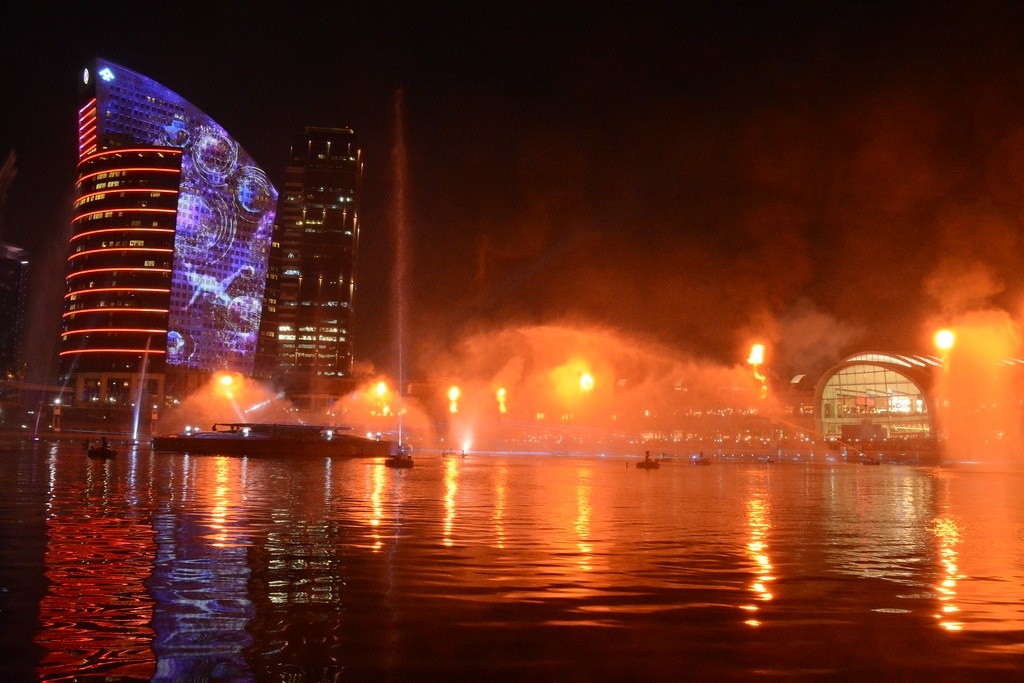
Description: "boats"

(692, 457), (711, 465)
(84, 439), (119, 456)
(758, 457), (774, 464)
(384, 445), (416, 469)
(860, 459), (880, 465)
(636, 450), (660, 469)
